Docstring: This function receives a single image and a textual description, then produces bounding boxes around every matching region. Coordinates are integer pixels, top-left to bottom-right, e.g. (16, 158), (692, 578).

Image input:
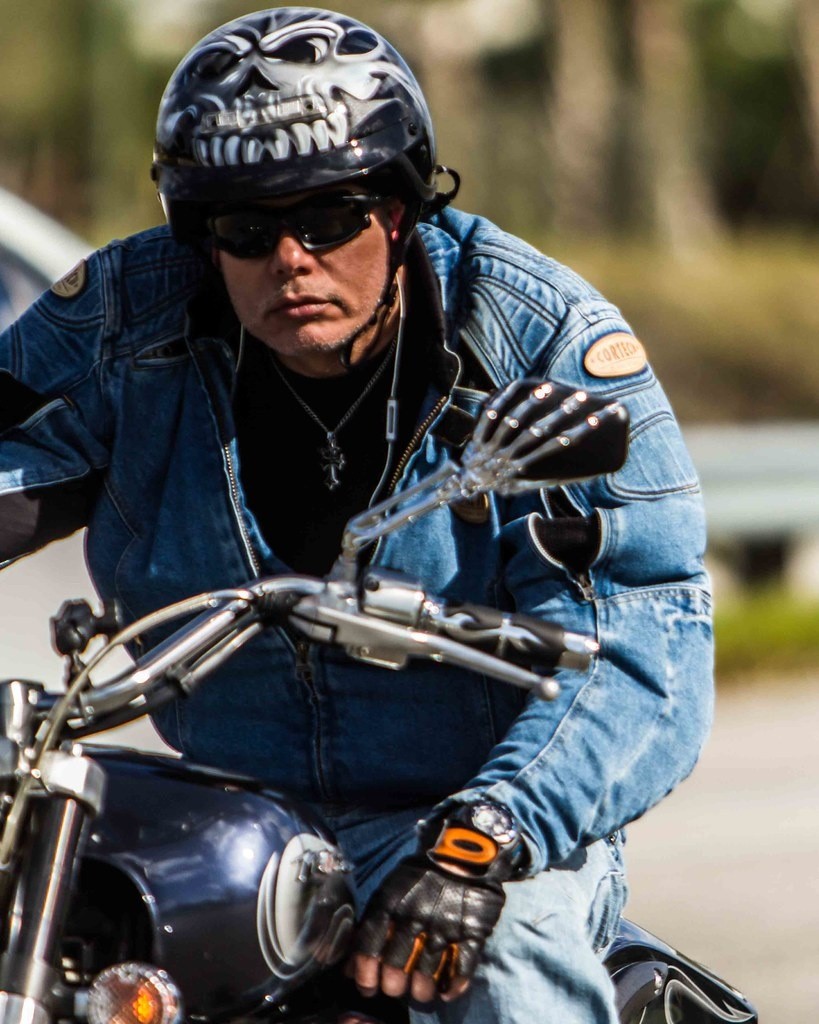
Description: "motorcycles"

(1, 377), (766, 1024)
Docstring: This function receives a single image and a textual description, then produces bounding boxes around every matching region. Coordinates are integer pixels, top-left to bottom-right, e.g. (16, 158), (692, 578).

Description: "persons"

(0, 5), (715, 1024)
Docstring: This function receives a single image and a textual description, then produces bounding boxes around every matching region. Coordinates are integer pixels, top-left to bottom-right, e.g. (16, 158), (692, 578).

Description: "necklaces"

(273, 349), (392, 491)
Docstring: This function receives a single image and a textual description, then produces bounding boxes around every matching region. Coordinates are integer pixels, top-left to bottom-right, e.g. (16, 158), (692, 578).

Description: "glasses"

(202, 187), (387, 261)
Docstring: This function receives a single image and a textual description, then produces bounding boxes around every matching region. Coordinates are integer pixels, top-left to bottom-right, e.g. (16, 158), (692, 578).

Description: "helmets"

(148, 5), (437, 248)
